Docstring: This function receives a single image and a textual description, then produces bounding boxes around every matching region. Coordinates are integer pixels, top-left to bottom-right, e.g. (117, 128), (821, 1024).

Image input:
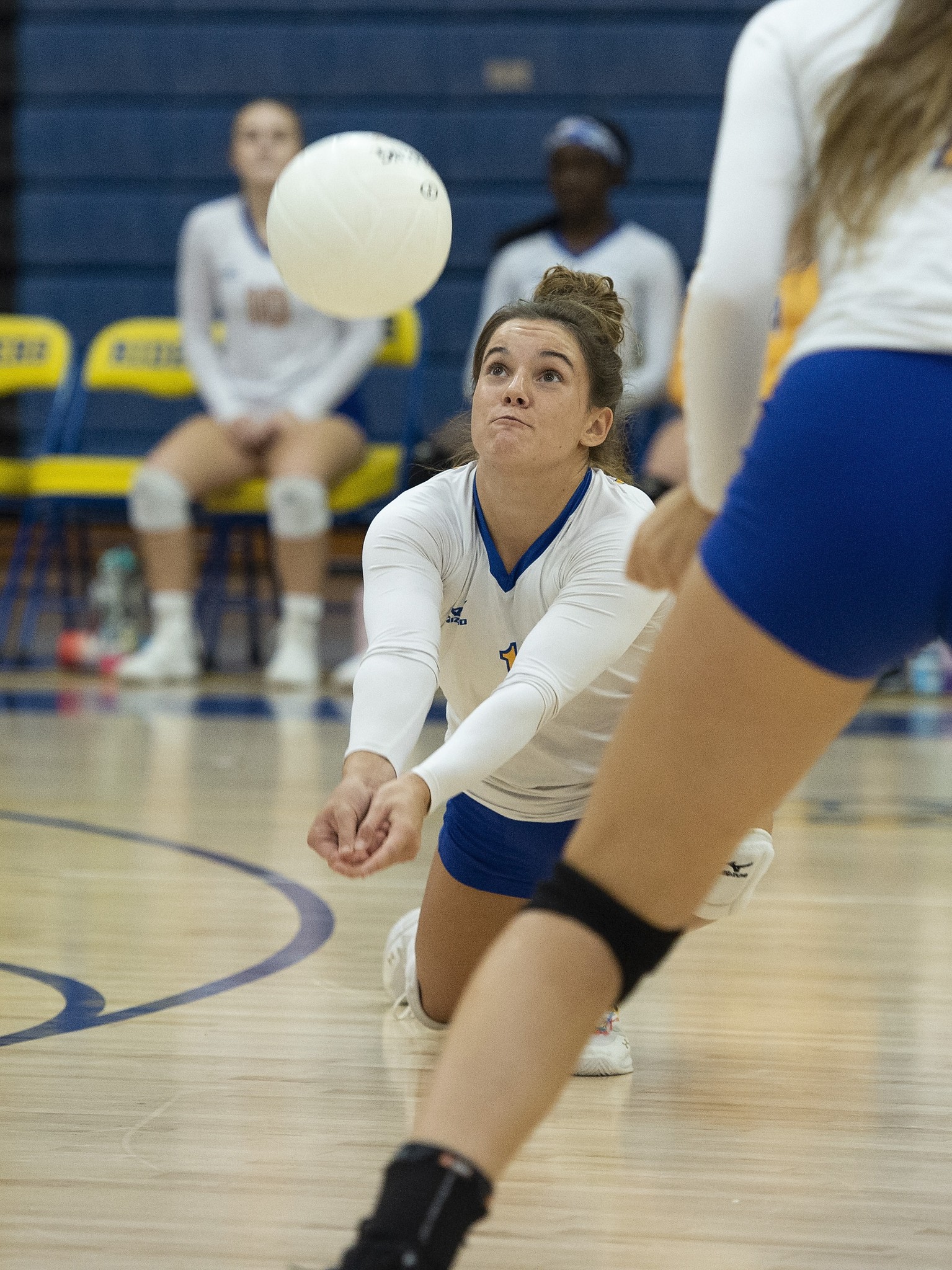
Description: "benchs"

(0, 0), (829, 533)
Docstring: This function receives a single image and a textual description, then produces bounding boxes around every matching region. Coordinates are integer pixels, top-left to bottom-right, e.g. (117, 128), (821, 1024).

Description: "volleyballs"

(265, 130), (454, 324)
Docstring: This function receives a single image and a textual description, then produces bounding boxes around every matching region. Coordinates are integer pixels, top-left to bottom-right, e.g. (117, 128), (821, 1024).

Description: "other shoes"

(118, 589), (198, 678)
(265, 595), (318, 686)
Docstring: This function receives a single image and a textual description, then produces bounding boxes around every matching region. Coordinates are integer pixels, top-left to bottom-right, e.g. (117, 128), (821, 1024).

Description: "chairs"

(207, 306), (438, 672)
(1, 303), (218, 675)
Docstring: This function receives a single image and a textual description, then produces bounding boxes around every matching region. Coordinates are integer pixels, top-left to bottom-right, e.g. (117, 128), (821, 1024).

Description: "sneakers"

(381, 906), (417, 1019)
(572, 1009), (634, 1075)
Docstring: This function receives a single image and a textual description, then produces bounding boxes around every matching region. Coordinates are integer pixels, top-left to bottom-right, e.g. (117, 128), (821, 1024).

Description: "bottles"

(910, 642), (942, 694)
(92, 548), (145, 674)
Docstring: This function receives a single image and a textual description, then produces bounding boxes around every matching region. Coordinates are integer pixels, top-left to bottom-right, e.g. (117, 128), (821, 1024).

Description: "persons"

(113, 99), (372, 686)
(308, 266), (775, 1076)
(337, 0), (952, 1270)
(465, 111), (679, 410)
